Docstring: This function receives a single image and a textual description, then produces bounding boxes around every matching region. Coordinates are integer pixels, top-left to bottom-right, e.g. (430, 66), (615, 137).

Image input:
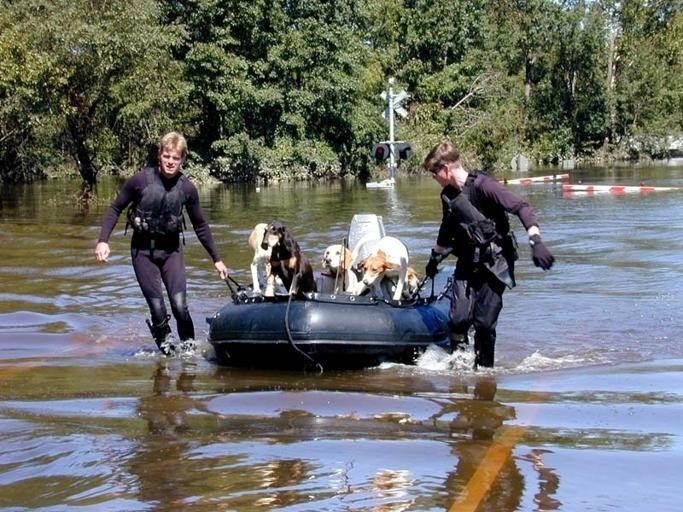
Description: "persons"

(93, 130), (229, 359)
(424, 142), (556, 367)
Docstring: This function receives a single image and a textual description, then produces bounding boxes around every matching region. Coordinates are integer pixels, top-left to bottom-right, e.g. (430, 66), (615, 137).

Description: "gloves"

(425, 249), (441, 280)
(529, 234), (555, 271)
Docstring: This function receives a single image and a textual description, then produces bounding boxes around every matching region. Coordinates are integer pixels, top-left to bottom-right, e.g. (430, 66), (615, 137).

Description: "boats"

(205, 264), (457, 373)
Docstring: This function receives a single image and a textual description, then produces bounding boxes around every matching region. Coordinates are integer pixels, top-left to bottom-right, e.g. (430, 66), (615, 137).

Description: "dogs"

(321, 244), (358, 295)
(261, 219), (318, 294)
(357, 236), (420, 306)
(248, 223), (284, 297)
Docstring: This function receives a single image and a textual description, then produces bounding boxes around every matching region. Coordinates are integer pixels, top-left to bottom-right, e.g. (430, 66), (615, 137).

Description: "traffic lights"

(400, 144), (412, 159)
(372, 143), (389, 160)
